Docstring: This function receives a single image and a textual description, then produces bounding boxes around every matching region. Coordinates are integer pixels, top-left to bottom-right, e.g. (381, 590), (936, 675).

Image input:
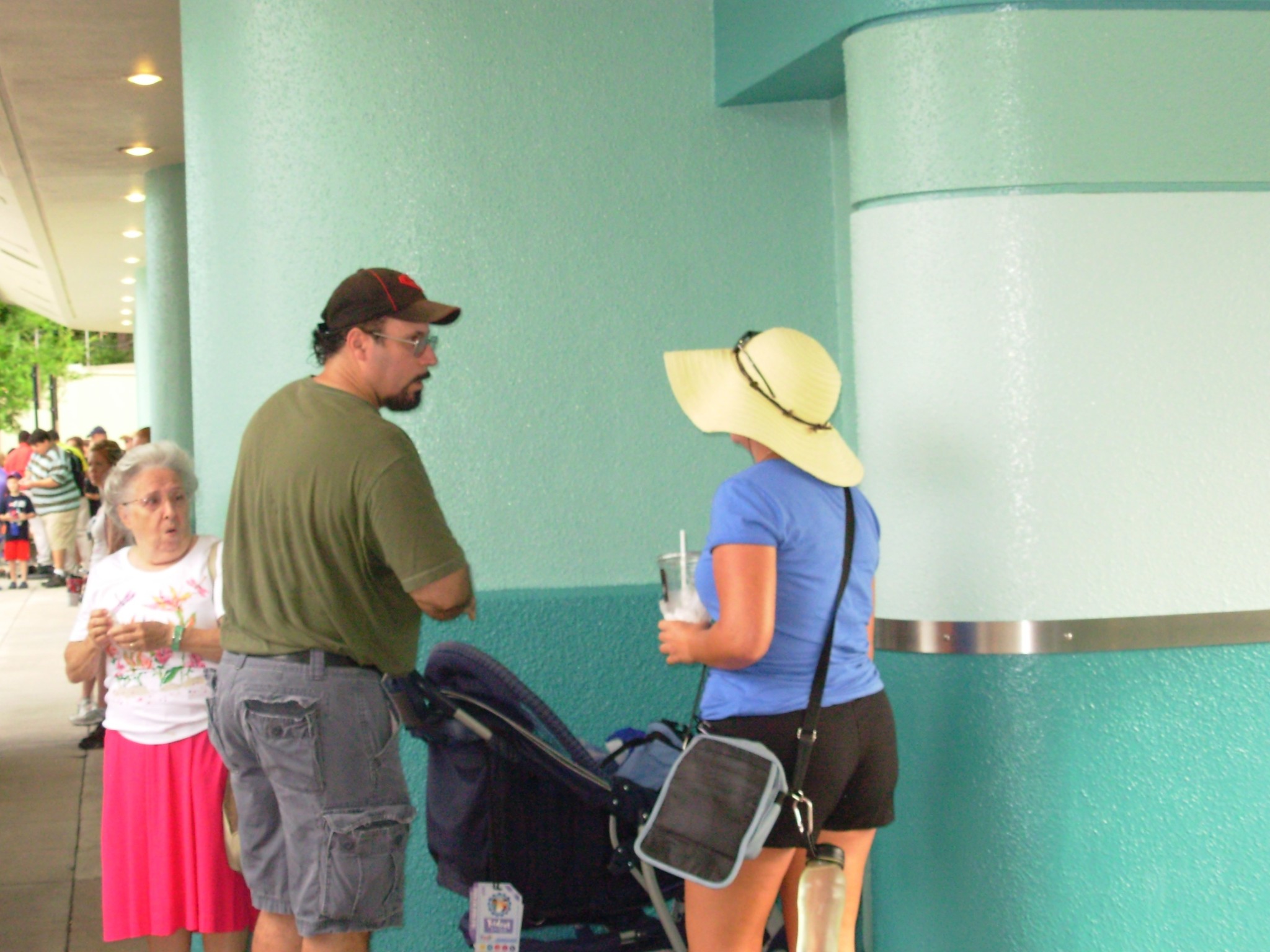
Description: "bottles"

(796, 843), (845, 952)
(10, 511), (19, 536)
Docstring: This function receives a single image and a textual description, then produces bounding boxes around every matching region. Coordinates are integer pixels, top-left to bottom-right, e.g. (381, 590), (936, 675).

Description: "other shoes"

(42, 574), (66, 588)
(69, 699), (104, 724)
(20, 583), (28, 589)
(78, 725), (106, 749)
(28, 565), (55, 579)
(9, 582), (16, 588)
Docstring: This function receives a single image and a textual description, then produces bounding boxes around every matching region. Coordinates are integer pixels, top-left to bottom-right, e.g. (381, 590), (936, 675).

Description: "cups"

(656, 550), (710, 630)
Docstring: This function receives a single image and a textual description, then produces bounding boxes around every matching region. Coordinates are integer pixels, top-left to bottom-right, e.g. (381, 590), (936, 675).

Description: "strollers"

(407, 639), (793, 952)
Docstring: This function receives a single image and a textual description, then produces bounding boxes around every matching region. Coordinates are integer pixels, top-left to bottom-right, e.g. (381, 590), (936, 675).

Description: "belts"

(274, 646), (382, 673)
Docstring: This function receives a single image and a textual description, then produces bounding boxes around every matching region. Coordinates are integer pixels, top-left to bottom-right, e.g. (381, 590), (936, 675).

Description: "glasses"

(119, 488), (190, 510)
(343, 325), (438, 357)
(733, 332), (775, 397)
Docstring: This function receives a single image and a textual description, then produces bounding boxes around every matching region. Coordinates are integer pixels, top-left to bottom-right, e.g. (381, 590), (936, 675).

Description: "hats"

(88, 426), (106, 437)
(322, 269), (461, 332)
(662, 327), (863, 488)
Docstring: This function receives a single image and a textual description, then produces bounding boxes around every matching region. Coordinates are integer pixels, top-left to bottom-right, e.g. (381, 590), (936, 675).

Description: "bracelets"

(170, 624), (183, 651)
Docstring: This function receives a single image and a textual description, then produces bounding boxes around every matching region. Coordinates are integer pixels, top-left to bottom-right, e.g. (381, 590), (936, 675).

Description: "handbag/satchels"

(632, 732), (785, 887)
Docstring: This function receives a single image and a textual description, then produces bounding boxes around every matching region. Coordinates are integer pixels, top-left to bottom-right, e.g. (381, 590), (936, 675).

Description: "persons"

(0, 426), (149, 750)
(657, 327), (901, 952)
(64, 439), (259, 951)
(206, 266), (478, 951)
